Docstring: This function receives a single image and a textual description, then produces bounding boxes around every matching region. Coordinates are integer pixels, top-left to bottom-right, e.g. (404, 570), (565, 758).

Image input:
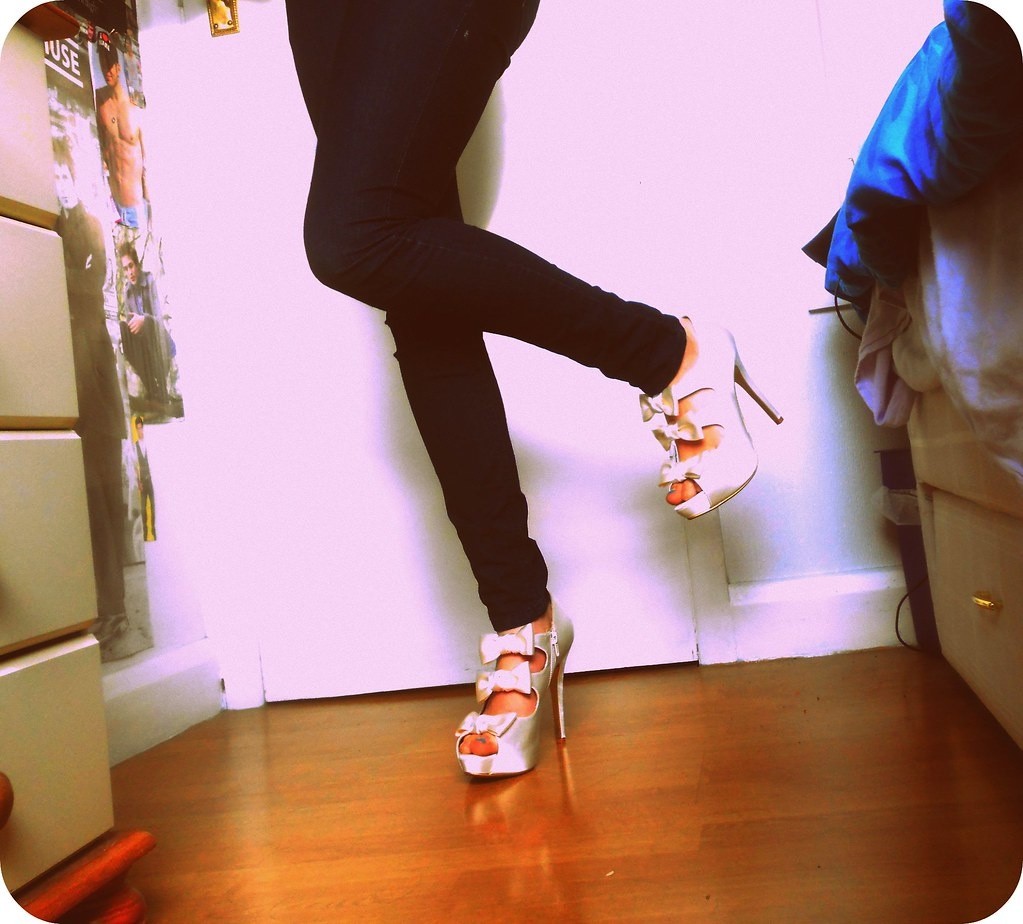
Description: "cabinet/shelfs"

(0, 3), (156, 923)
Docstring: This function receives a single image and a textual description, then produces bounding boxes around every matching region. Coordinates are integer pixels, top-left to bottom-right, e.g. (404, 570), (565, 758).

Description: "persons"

(119, 244), (176, 402)
(133, 416), (156, 541)
(94, 31), (152, 233)
(285, 0), (782, 778)
(53, 136), (127, 647)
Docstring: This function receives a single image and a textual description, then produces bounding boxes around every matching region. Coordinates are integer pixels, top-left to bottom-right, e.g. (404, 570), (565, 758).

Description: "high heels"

(639, 315), (785, 522)
(454, 597), (575, 778)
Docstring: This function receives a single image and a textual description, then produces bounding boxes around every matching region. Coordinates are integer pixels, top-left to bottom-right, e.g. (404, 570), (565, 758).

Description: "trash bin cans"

(875, 447), (943, 653)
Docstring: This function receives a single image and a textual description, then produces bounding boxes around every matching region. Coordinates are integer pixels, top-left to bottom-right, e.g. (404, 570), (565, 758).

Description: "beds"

(811, 1), (1023, 776)
